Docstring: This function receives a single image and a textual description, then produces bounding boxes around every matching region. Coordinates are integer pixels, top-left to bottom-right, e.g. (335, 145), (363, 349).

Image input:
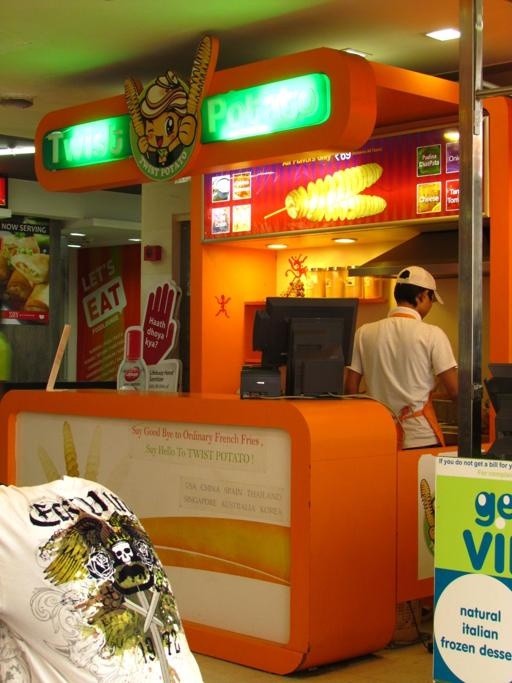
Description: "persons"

(343, 265), (459, 450)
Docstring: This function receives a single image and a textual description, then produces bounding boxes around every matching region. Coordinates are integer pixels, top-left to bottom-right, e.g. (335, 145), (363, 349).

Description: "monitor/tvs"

(252, 296), (360, 400)
(484, 362), (512, 459)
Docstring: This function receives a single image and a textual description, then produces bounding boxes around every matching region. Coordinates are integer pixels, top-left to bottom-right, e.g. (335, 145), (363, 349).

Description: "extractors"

(346, 227), (491, 277)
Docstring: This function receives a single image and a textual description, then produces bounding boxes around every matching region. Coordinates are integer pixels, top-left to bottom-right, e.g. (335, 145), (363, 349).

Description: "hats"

(397, 266), (443, 303)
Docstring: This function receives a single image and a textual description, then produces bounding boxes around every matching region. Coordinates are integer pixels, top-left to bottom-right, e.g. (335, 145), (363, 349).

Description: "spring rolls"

(0, 233), (49, 313)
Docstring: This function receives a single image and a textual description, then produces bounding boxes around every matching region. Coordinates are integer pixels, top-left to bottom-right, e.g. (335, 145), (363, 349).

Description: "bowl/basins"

(216, 177), (229, 199)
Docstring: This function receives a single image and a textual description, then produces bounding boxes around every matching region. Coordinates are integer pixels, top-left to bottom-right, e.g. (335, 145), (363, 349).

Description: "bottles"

(305, 266), (385, 301)
(117, 329), (150, 390)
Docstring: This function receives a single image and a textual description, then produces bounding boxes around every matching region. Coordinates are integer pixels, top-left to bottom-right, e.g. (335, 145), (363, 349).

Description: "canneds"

(309, 265), (391, 299)
